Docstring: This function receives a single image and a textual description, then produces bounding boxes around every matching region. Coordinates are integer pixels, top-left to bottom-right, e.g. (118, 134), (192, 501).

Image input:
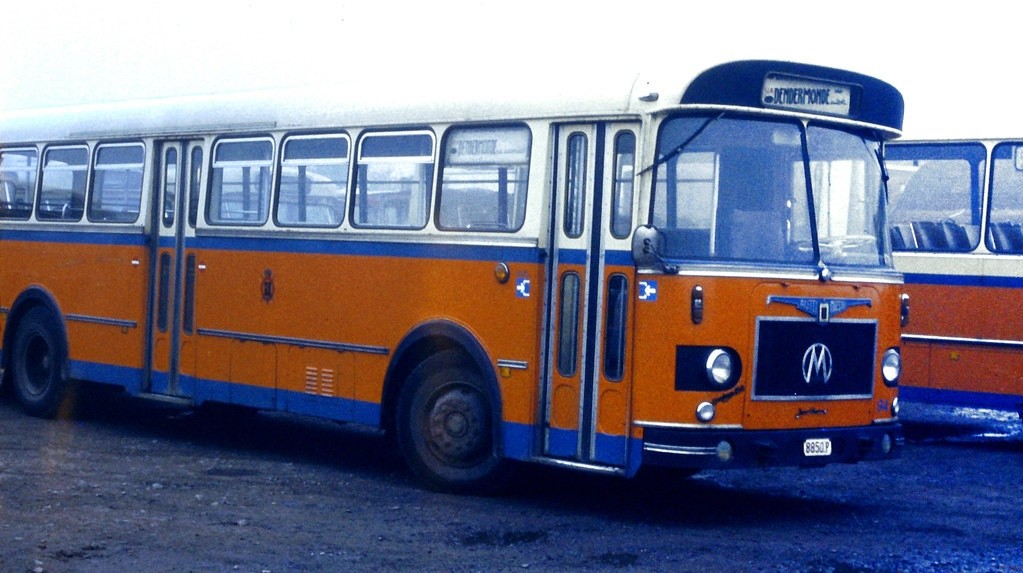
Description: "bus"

(621, 136), (1023, 445)
(0, 54), (905, 494)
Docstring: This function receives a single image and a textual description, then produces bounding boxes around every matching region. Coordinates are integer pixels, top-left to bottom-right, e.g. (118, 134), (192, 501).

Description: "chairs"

(886, 220), (1023, 254)
(727, 208), (784, 262)
